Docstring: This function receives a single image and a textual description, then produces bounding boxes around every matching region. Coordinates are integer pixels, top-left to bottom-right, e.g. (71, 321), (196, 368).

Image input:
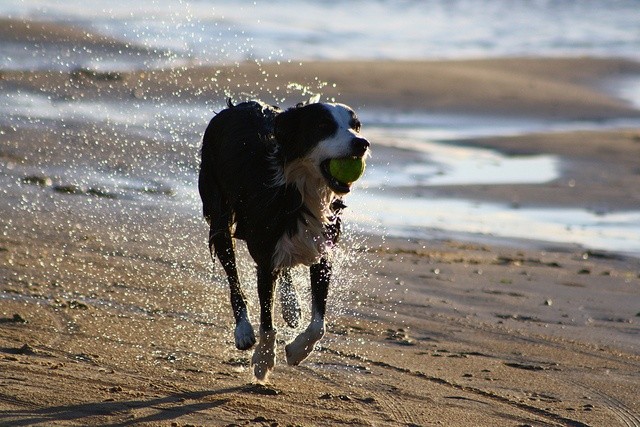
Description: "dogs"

(199, 97), (370, 382)
